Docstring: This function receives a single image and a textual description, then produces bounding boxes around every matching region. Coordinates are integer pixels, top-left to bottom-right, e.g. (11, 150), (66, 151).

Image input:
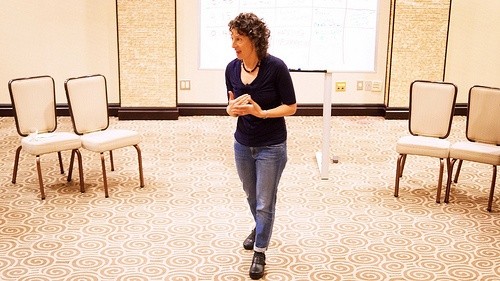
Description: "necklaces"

(242, 61), (260, 73)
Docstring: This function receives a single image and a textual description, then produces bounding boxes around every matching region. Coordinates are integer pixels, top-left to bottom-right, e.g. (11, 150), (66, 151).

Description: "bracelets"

(263, 110), (268, 119)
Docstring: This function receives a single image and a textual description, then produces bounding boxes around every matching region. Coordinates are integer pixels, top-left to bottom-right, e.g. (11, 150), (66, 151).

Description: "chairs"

(394, 79), (458, 205)
(8, 75), (85, 200)
(64, 74), (144, 198)
(445, 84), (500, 212)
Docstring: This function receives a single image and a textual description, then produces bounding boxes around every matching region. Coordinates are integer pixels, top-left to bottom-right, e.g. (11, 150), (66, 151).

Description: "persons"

(225, 12), (297, 280)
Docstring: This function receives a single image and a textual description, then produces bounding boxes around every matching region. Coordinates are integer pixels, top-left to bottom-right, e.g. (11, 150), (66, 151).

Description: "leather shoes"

(243, 227), (256, 250)
(249, 253), (266, 279)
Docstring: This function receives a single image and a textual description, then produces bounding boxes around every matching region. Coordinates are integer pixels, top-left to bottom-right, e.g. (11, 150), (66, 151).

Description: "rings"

(242, 111), (245, 115)
